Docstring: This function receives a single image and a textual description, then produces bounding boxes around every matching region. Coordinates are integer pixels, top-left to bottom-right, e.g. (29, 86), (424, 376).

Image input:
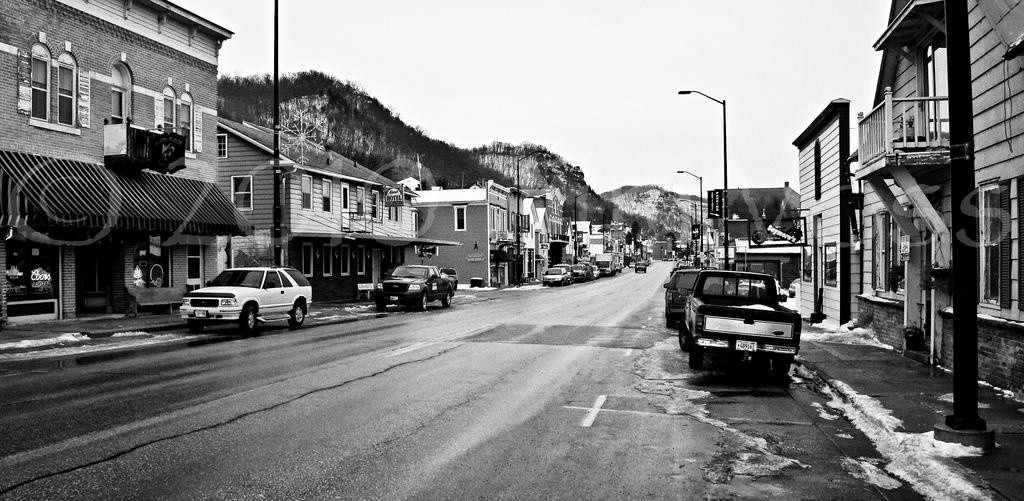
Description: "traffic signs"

(707, 189), (724, 219)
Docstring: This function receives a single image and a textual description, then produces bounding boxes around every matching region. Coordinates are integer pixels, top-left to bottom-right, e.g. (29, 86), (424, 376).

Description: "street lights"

(574, 190), (630, 257)
(674, 90), (730, 272)
(516, 149), (550, 287)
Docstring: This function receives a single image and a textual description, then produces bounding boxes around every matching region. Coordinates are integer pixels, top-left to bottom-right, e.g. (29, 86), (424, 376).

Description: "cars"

(179, 266), (312, 331)
(543, 253), (651, 287)
(375, 265), (458, 311)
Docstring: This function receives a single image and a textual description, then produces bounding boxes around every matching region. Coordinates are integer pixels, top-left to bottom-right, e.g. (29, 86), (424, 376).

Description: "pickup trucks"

(664, 266), (802, 375)
